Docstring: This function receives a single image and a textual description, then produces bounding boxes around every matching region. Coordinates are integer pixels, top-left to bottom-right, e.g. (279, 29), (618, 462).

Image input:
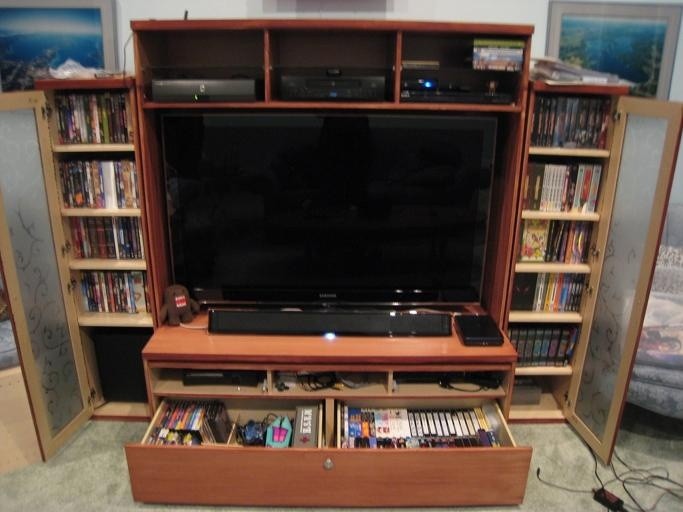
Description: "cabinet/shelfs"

(0, 74), (158, 463)
(503, 85), (683, 466)
(128, 19), (534, 507)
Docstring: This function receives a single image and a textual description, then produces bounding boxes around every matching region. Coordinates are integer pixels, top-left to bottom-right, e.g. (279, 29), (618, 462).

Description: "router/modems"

(454, 314), (504, 346)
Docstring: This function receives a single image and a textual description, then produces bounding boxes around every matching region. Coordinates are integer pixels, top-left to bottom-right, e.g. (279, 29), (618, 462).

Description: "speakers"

(95, 332), (150, 402)
(208, 310), (451, 336)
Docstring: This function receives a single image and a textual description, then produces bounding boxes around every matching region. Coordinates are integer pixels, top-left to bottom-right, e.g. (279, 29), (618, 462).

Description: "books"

(402, 59), (439, 71)
(55, 94), (150, 313)
(148, 399), (230, 450)
(334, 400), (500, 447)
(473, 37), (628, 86)
(508, 91), (611, 369)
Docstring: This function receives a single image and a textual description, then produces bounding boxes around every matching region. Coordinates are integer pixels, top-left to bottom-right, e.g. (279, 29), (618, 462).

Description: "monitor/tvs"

(157, 110), (513, 307)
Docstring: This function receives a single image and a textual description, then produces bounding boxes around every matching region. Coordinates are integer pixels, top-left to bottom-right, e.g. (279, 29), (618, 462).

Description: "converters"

(594, 489), (623, 511)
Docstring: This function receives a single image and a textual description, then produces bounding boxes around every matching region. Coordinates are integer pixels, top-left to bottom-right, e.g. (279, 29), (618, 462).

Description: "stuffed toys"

(159, 284), (199, 326)
